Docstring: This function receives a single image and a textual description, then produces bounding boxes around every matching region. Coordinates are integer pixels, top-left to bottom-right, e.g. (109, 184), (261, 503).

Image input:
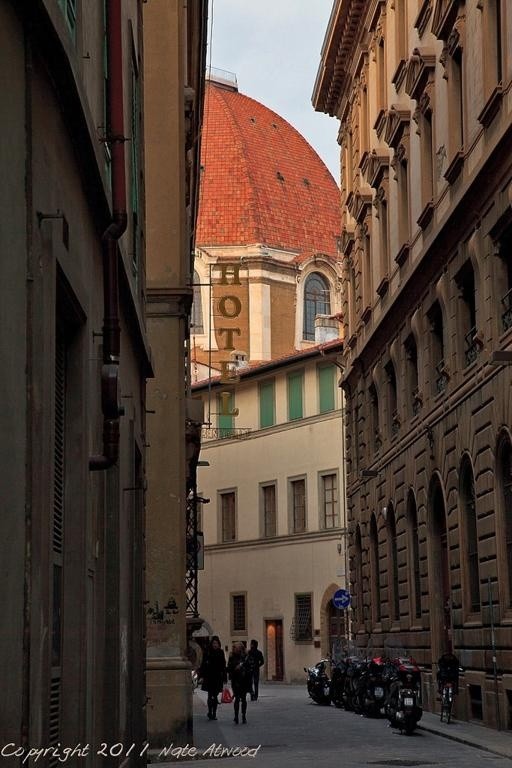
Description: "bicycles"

(429, 662), (467, 724)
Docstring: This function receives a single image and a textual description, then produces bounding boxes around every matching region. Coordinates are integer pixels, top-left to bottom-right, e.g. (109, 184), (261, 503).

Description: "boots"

(234, 703), (247, 724)
(207, 699), (217, 720)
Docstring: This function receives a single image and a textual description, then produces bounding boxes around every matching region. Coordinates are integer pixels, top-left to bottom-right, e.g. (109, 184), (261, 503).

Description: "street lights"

(485, 348), (512, 366)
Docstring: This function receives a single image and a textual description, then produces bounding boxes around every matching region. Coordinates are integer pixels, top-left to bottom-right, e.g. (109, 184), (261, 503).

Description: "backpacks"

(221, 688), (232, 703)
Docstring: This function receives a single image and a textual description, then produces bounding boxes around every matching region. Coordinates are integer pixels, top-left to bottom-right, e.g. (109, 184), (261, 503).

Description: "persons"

(436, 646), (459, 695)
(193, 636), (265, 723)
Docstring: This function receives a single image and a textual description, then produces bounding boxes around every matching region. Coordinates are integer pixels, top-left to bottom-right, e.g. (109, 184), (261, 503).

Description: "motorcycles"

(301, 652), (425, 738)
